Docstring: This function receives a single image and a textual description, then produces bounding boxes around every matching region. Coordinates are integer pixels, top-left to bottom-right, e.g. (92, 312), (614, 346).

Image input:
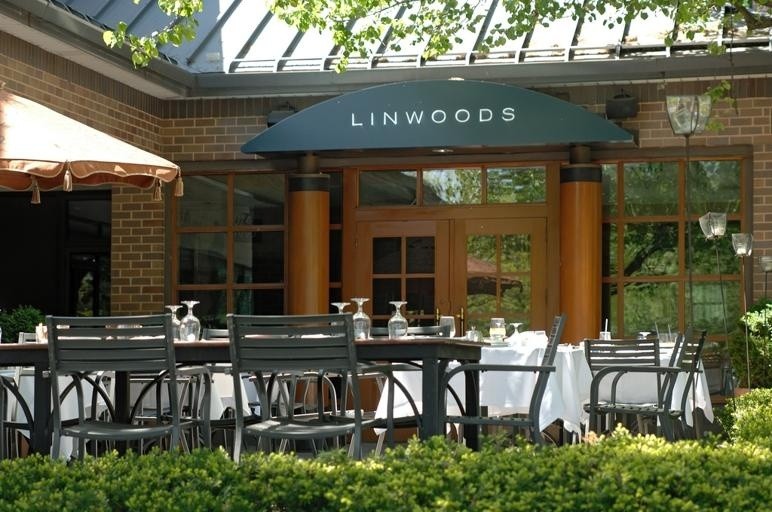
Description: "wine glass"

(489, 317), (506, 342)
(386, 301), (408, 339)
(350, 297), (372, 340)
(164, 299), (201, 343)
(331, 302), (350, 337)
(439, 317), (456, 338)
(510, 322), (525, 335)
(638, 331), (651, 347)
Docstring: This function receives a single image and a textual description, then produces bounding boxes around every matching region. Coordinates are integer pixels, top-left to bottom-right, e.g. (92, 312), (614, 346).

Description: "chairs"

(0, 312), (714, 466)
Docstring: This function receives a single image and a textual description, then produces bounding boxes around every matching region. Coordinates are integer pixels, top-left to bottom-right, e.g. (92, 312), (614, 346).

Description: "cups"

(466, 330), (482, 342)
(658, 334), (679, 343)
(599, 331), (611, 353)
